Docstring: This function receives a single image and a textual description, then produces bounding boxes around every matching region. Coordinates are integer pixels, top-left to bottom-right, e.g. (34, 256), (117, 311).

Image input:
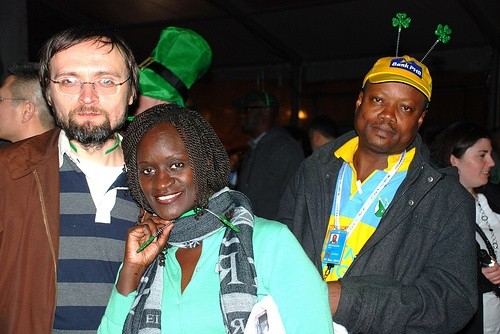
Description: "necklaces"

(477, 200), (498, 256)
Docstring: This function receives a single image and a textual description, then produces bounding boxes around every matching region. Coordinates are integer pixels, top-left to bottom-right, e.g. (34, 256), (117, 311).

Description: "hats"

(136, 26), (212, 109)
(360, 55), (433, 103)
(246, 88), (275, 107)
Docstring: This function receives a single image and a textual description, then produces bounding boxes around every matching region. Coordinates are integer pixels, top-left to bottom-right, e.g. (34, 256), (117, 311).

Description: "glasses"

(48, 73), (133, 96)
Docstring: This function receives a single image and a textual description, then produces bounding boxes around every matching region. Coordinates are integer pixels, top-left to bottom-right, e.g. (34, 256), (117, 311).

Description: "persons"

(308, 112), (338, 150)
(274, 12), (478, 334)
(441, 122), (500, 334)
(227, 148), (240, 169)
(236, 90), (305, 222)
(121, 26), (212, 131)
(96, 103), (335, 334)
(0, 61), (57, 148)
(0, 26), (139, 334)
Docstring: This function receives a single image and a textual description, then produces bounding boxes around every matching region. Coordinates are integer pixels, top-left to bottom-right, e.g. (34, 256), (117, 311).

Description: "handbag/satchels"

(475, 223), (500, 299)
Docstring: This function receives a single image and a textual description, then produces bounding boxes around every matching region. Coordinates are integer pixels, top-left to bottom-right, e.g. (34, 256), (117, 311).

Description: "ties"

(244, 147), (256, 185)
